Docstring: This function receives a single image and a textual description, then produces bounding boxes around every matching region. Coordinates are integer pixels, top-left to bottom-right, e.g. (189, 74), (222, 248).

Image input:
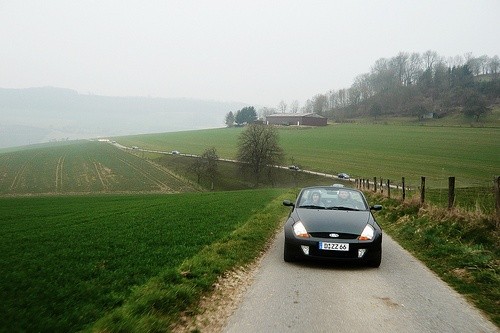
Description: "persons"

(325, 188), (360, 209)
(303, 189), (324, 207)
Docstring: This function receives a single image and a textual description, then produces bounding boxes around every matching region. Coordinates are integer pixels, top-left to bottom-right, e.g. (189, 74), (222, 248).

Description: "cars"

(282, 186), (383, 268)
